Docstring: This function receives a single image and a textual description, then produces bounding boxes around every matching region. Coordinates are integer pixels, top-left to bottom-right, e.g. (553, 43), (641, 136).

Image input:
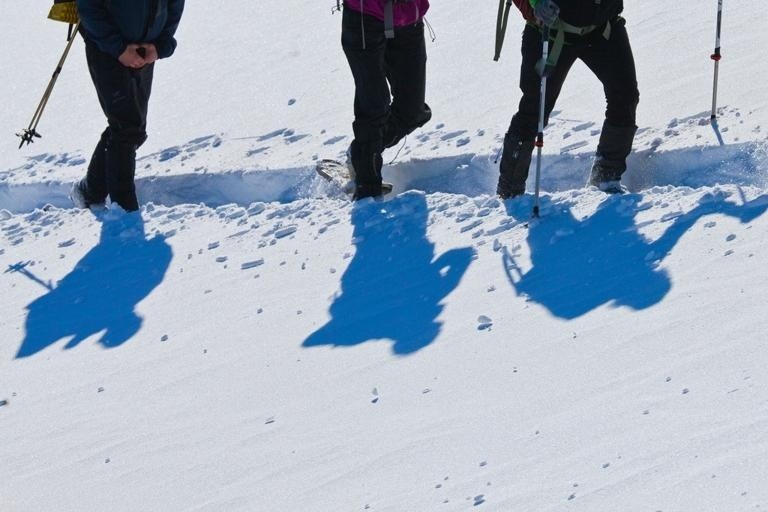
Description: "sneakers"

(350, 182), (393, 199)
(68, 180), (110, 210)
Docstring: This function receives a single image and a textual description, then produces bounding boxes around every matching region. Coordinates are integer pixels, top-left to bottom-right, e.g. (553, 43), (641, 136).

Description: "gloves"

(534, 1), (560, 24)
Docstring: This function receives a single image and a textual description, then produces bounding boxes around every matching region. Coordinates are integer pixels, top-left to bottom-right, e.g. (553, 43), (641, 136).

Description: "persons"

(68, 0), (186, 212)
(496, 0), (641, 200)
(341, 0), (431, 202)
